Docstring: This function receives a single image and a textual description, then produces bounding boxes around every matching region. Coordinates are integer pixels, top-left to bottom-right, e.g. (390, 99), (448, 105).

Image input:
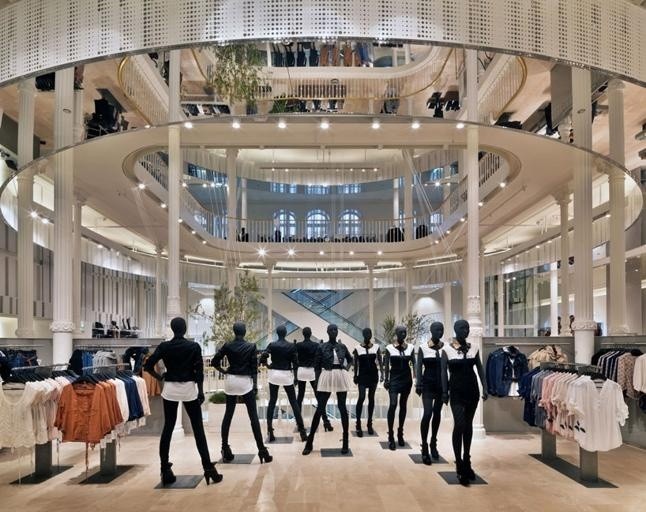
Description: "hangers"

(1, 364), (75, 395)
(73, 365), (143, 393)
(601, 350), (641, 364)
(79, 344), (127, 354)
(540, 346), (572, 361)
(538, 368), (618, 392)
(496, 348), (528, 368)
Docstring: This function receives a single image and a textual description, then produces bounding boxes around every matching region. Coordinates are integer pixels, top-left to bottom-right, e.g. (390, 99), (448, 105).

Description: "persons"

(302, 324), (353, 455)
(211, 322), (273, 463)
(352, 328), (384, 438)
(440, 320), (489, 485)
(384, 327), (418, 450)
(296, 328), (333, 432)
(259, 326), (308, 442)
(144, 317), (223, 486)
(416, 322), (450, 465)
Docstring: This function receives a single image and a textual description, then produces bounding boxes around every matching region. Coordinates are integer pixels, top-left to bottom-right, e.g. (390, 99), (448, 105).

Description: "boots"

(161, 462), (176, 488)
(299, 428), (308, 441)
(258, 446), (273, 464)
(453, 459), (470, 487)
(267, 428), (275, 443)
(202, 462), (223, 485)
(430, 438), (439, 460)
(463, 454), (476, 482)
(356, 421), (363, 437)
(323, 420), (333, 432)
(387, 430), (396, 450)
(367, 420), (374, 435)
(341, 432), (349, 454)
(397, 427), (405, 446)
(221, 445), (234, 463)
(302, 436), (313, 455)
(420, 443), (432, 466)
